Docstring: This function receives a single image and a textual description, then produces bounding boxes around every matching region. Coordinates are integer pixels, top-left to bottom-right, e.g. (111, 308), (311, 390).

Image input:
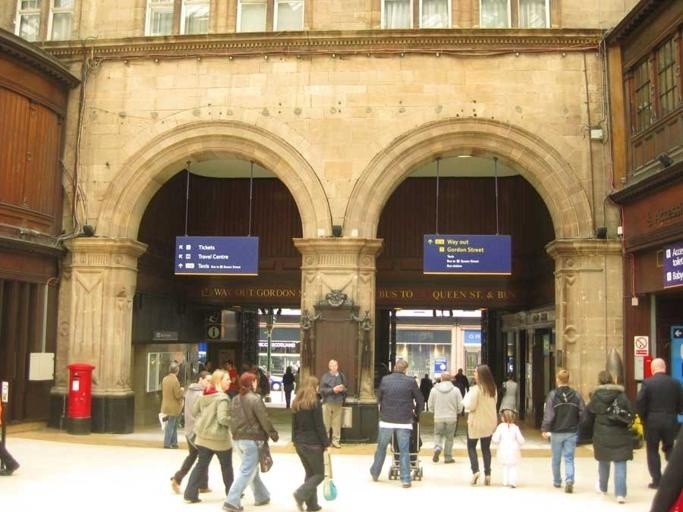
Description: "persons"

(222, 371), (279, 512)
(460, 364), (500, 486)
(290, 376), (332, 512)
(417, 372), (433, 411)
(0, 394), (21, 476)
(486, 408), (527, 489)
(160, 365), (186, 449)
(368, 358), (426, 488)
(648, 419), (683, 512)
(222, 360), (239, 399)
(454, 368), (470, 398)
(631, 357), (682, 490)
(318, 359), (349, 448)
(428, 371), (463, 465)
(587, 370), (637, 504)
(282, 366), (295, 409)
(539, 369), (587, 494)
(500, 372), (520, 416)
(169, 371), (215, 495)
(183, 368), (245, 503)
(250, 365), (270, 400)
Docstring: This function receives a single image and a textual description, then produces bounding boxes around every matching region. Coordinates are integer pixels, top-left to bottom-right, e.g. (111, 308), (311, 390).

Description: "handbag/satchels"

(323, 480), (336, 500)
(284, 383), (294, 393)
(606, 392), (635, 427)
(577, 404), (594, 439)
(497, 388), (507, 401)
(159, 413), (168, 433)
(258, 442), (273, 472)
(192, 397), (229, 440)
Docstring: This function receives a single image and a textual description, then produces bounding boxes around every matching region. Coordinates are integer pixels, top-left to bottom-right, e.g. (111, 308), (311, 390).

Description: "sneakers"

(307, 505), (322, 512)
(172, 481), (271, 507)
(431, 448), (454, 464)
(294, 492), (305, 512)
(404, 483), (411, 488)
(0, 459), (19, 476)
(370, 468), (379, 481)
(469, 475), (662, 504)
(331, 441), (341, 448)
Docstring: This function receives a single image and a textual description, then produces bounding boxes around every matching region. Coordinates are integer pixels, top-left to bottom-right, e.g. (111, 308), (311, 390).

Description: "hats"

(499, 403), (517, 415)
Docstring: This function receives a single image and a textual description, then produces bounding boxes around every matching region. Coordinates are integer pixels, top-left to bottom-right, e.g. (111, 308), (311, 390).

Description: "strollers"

(387, 408), (422, 482)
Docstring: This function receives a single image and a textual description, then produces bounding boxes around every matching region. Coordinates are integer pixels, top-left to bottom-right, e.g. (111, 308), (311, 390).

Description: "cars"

(260, 367), (283, 391)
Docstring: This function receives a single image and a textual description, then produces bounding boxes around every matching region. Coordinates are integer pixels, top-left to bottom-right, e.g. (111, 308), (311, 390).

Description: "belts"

(649, 409), (678, 413)
